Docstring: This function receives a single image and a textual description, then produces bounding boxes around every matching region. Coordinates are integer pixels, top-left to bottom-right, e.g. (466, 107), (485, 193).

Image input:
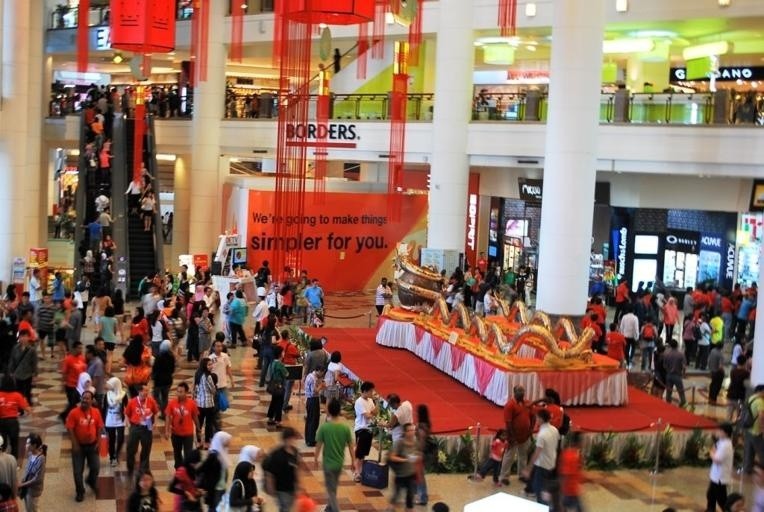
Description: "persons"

(333, 49), (343, 73)
(440, 250), (534, 317)
(580, 275), (758, 512)
(54, 213), (62, 238)
(1, 262), (451, 511)
(77, 190), (117, 260)
(124, 161), (160, 234)
(475, 384), (595, 511)
(49, 79), (182, 119)
(82, 115), (116, 195)
(223, 88), (262, 120)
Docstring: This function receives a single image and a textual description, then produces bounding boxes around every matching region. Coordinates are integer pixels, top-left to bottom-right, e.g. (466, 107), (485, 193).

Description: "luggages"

(361, 459), (389, 489)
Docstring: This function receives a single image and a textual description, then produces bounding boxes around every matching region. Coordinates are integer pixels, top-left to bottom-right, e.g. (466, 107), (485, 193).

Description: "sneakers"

(469, 470), (537, 497)
(352, 470), (364, 483)
(85, 477), (99, 495)
(107, 455), (120, 467)
(75, 486), (85, 502)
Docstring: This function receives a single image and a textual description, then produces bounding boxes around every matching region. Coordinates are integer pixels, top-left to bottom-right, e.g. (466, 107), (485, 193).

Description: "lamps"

(526, 4), (535, 17)
(386, 11), (395, 25)
(616, 0), (627, 12)
(718, 0), (730, 6)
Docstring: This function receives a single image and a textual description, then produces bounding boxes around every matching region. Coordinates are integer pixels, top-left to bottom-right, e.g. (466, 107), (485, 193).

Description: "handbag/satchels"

(281, 341), (303, 380)
(266, 379), (286, 397)
(214, 478), (249, 511)
(16, 480), (28, 499)
(217, 388), (231, 412)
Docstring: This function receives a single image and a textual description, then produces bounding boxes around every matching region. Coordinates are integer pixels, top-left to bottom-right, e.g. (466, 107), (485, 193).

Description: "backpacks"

(423, 432), (439, 455)
(642, 325), (655, 341)
(692, 322), (706, 338)
(740, 404), (753, 429)
